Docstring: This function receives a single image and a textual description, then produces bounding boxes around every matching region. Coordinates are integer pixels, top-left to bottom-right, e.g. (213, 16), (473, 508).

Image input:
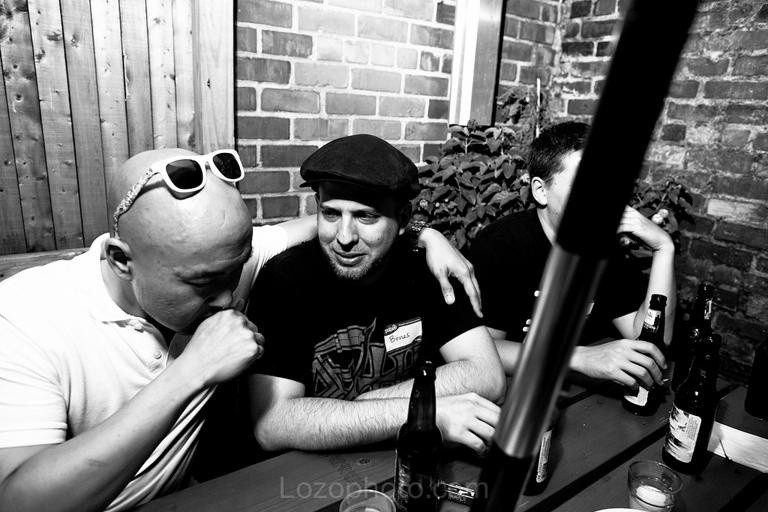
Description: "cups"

(339, 488), (397, 511)
(625, 460), (683, 512)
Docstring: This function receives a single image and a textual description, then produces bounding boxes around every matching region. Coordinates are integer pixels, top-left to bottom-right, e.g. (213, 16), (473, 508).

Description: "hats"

(298, 134), (421, 199)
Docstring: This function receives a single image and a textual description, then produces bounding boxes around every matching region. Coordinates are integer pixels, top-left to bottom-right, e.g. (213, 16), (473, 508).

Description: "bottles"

(522, 427), (555, 497)
(391, 360), (443, 512)
(670, 284), (718, 394)
(619, 294), (668, 418)
(660, 329), (724, 476)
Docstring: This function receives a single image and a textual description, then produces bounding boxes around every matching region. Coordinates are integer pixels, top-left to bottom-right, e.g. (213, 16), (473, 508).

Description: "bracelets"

(403, 218), (424, 246)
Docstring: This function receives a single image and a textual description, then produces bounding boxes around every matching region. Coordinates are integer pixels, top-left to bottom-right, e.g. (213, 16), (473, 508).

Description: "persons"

(0, 145), (485, 511)
(216, 133), (507, 458)
(461, 120), (676, 393)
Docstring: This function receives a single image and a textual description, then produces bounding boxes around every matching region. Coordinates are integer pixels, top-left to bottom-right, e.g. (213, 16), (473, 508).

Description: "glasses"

(113, 148), (246, 239)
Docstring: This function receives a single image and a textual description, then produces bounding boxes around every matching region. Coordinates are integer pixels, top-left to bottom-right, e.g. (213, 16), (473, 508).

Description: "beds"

(128, 356), (768, 512)
